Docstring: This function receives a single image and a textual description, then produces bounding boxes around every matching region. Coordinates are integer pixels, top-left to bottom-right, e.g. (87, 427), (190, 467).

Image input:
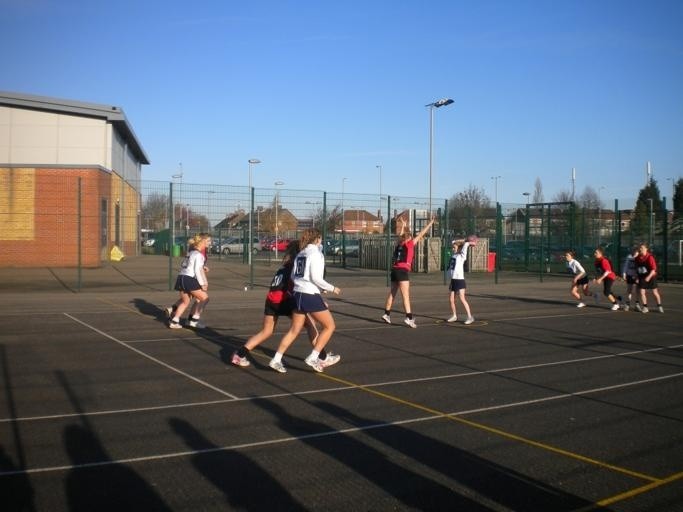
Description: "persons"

(168, 235), (208, 331)
(164, 233), (211, 325)
(445, 237), (477, 325)
(229, 239), (341, 368)
(380, 215), (435, 329)
(267, 228), (342, 373)
(592, 248), (623, 312)
(636, 243), (665, 314)
(565, 249), (600, 309)
(623, 246), (642, 313)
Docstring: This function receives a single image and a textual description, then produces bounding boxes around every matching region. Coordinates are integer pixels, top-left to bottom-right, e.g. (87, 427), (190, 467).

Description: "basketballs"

(467, 235), (478, 246)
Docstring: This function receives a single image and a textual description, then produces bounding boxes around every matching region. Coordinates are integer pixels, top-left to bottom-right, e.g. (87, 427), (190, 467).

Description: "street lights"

(247, 160), (261, 262)
(172, 174), (184, 237)
(376, 165), (383, 215)
(522, 192), (530, 203)
(646, 197), (654, 245)
(273, 181), (285, 259)
(491, 174), (501, 208)
(340, 177), (348, 236)
(424, 99), (454, 237)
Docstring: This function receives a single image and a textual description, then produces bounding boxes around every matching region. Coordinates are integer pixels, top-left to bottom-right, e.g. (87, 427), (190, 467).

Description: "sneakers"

(229, 353), (250, 366)
(447, 316), (457, 322)
(305, 354), (341, 372)
(611, 296), (648, 313)
(658, 306), (663, 313)
(268, 359), (287, 373)
(382, 314), (391, 324)
(164, 307), (204, 329)
(577, 303), (586, 308)
(464, 317), (474, 324)
(595, 294), (600, 303)
(403, 317), (416, 328)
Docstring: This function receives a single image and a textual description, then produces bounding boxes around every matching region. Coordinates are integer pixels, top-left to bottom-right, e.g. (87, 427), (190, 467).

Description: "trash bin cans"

(488, 252), (496, 272)
(173, 246), (181, 257)
(440, 247), (452, 271)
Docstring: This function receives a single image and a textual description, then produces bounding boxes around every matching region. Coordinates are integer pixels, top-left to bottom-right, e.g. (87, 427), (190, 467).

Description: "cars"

(489, 241), (674, 262)
(143, 235), (361, 257)
